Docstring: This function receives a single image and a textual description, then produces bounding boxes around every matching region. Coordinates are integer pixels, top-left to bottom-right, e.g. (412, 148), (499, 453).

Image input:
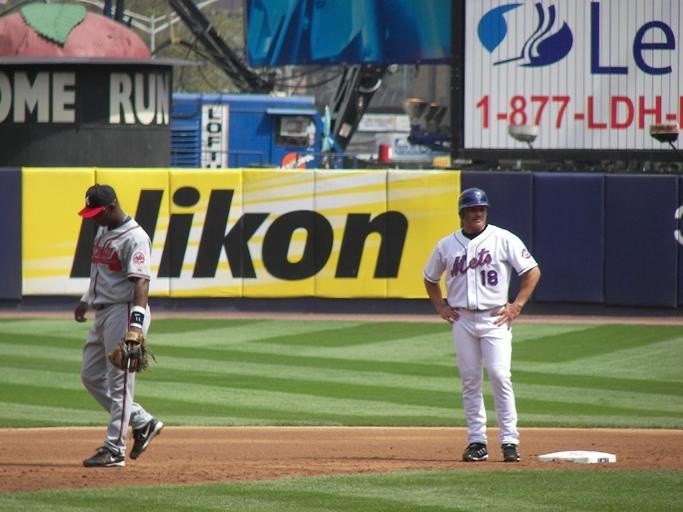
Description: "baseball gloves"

(108, 331), (151, 371)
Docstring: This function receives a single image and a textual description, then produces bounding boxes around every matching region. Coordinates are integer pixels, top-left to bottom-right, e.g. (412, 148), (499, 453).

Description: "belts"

(95, 302), (111, 311)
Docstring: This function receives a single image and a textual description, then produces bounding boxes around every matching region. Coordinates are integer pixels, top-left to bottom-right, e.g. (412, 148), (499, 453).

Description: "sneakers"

(501, 444), (520, 462)
(129, 418), (163, 459)
(83, 448), (124, 467)
(461, 443), (488, 462)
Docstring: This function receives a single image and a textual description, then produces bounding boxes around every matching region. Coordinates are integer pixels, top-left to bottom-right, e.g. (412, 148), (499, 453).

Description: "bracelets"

(129, 306), (146, 328)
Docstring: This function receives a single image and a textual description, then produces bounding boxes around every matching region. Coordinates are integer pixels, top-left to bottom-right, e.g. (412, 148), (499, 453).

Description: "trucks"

(170, 93), (357, 169)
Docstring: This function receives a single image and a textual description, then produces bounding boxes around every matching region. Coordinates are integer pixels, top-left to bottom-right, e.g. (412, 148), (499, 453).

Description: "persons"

(74, 184), (165, 467)
(424, 187), (541, 462)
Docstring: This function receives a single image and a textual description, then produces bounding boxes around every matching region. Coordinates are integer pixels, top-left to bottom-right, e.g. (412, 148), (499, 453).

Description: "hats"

(79, 182), (115, 217)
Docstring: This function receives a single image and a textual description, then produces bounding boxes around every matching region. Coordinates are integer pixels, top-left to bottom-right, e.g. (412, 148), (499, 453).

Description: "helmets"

(459, 187), (490, 210)
